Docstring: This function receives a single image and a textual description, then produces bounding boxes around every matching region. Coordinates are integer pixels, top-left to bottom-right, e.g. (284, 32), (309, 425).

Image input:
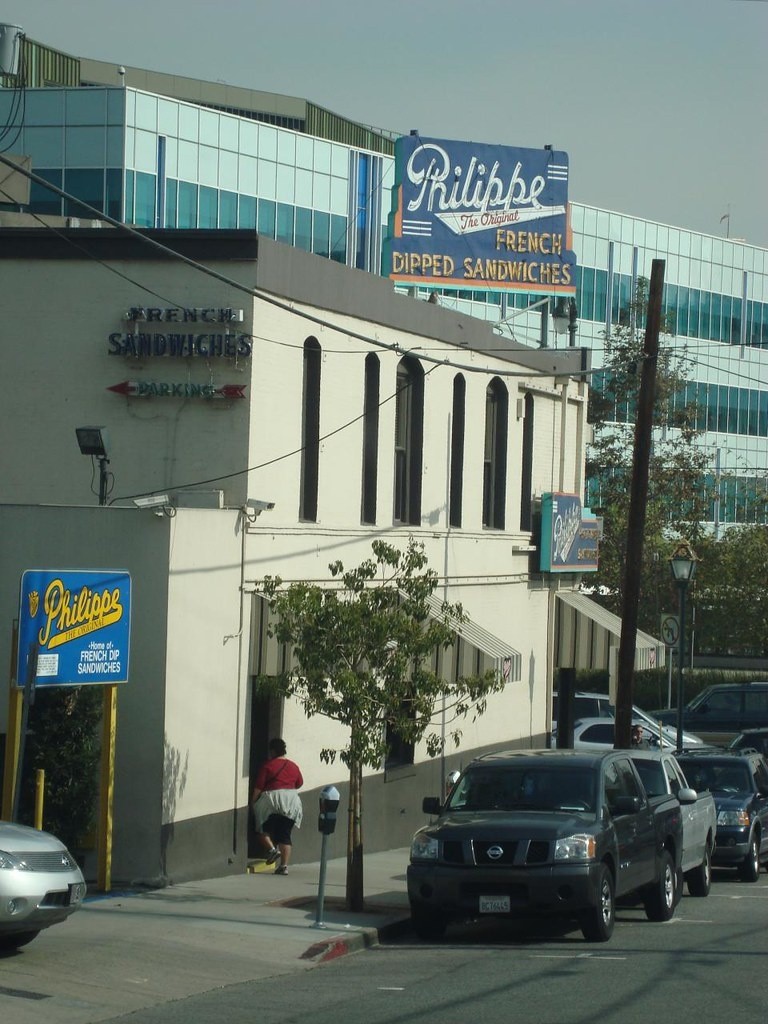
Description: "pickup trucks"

(408, 749), (683, 942)
(615, 749), (717, 898)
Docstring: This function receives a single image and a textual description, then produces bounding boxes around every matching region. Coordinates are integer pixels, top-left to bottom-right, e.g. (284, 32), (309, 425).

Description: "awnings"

(397, 588), (522, 683)
(556, 593), (666, 671)
(255, 591), (398, 654)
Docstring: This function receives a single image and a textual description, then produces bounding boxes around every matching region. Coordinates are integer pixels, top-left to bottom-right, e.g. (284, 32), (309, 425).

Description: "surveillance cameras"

(246, 499), (276, 511)
(132, 494), (169, 509)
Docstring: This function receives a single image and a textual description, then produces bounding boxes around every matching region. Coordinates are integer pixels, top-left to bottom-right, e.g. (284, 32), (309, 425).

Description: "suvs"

(672, 747), (768, 884)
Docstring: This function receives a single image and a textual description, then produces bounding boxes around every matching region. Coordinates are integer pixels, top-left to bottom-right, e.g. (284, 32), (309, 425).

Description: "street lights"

(668, 539), (701, 769)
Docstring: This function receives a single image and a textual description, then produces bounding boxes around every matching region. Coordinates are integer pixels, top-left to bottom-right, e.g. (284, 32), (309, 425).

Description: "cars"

(553, 691), (703, 744)
(550, 717), (722, 752)
(641, 681), (768, 747)
(0, 821), (87, 950)
(723, 727), (768, 758)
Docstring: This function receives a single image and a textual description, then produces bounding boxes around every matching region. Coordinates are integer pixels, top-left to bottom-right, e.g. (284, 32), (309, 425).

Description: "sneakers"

(274, 866), (288, 875)
(266, 849), (280, 865)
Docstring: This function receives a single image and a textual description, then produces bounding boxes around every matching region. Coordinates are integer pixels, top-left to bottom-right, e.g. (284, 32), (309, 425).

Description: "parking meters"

(310, 786), (341, 931)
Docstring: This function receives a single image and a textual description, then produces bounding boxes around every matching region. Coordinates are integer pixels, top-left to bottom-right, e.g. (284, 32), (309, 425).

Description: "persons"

(631, 725), (650, 751)
(251, 738), (303, 875)
